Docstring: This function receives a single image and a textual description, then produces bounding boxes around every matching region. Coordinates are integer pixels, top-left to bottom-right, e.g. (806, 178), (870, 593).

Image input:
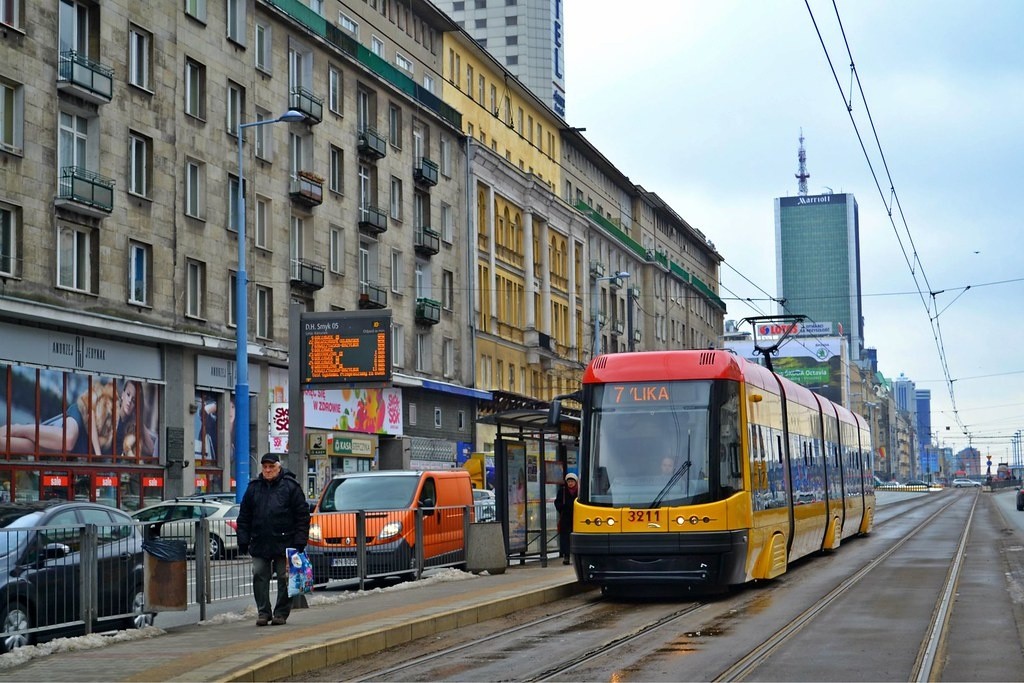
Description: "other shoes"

(255, 615), (272, 627)
(272, 617), (287, 626)
(562, 555), (570, 565)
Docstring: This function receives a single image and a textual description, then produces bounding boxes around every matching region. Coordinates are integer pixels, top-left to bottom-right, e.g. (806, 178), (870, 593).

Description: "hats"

(565, 473), (578, 482)
(261, 453), (280, 464)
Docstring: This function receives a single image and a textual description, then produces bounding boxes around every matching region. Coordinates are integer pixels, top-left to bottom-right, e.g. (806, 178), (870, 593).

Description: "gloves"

(293, 545), (305, 552)
(239, 545), (248, 555)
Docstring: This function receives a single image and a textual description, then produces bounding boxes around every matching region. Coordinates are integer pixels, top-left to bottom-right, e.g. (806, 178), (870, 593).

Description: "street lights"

(235, 108), (307, 510)
(593, 272), (631, 360)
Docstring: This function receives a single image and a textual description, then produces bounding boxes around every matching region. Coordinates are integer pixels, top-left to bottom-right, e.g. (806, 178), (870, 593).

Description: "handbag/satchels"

(558, 510), (569, 532)
(285, 546), (315, 599)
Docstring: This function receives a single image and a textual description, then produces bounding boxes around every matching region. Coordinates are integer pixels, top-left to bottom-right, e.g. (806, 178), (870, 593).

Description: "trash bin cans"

(468, 522), (508, 574)
(142, 541), (189, 611)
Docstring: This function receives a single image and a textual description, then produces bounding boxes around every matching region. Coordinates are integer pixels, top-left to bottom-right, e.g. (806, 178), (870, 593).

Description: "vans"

(310, 470), (476, 583)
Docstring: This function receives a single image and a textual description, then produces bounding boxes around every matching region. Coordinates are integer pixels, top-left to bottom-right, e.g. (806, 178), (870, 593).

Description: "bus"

(545, 350), (877, 598)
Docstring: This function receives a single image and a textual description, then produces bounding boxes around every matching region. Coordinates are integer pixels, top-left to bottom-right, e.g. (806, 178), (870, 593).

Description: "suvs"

(130, 492), (241, 560)
(952, 478), (982, 488)
(472, 488), (495, 523)
(0, 499), (159, 656)
(905, 480), (932, 488)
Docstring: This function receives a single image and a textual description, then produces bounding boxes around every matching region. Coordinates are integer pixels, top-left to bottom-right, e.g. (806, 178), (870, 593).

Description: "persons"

(554, 473), (578, 564)
(236, 453), (310, 626)
(0, 380), (237, 465)
